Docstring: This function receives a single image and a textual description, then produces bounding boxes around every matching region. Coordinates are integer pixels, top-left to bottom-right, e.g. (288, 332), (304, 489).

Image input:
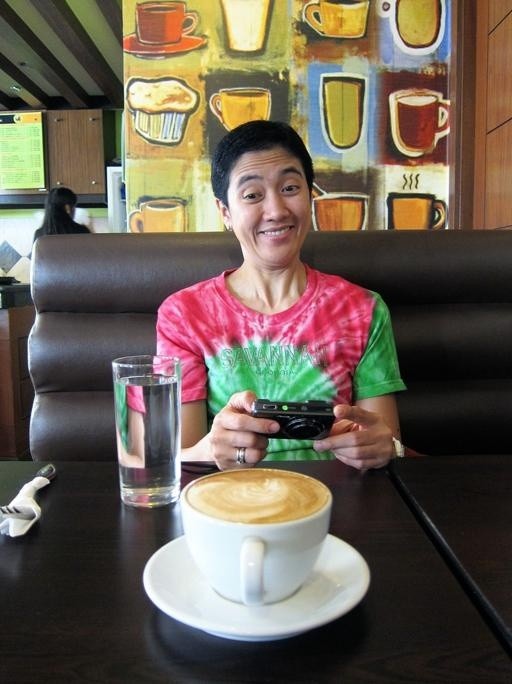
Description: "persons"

(113, 378), (147, 469)
(34, 186), (91, 238)
(156, 120), (410, 473)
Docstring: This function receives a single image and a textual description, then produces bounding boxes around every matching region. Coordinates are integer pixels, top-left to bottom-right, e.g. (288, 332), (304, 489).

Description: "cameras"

(251, 399), (336, 440)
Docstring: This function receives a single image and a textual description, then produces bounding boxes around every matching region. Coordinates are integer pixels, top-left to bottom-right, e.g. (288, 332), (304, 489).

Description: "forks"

(2, 461), (58, 522)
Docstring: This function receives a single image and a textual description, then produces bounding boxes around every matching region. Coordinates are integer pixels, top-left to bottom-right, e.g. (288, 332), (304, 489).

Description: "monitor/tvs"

(0, 109), (50, 195)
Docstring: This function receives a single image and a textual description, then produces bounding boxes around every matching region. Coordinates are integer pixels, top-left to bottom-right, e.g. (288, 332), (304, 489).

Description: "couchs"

(27, 230), (512, 462)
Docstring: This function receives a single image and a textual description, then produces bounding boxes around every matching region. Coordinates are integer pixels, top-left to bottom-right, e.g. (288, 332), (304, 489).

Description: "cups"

(112, 355), (184, 509)
(182, 468), (333, 608)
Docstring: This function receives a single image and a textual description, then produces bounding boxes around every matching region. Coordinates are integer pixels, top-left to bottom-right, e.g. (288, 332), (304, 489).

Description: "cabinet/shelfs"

(0, 109), (116, 209)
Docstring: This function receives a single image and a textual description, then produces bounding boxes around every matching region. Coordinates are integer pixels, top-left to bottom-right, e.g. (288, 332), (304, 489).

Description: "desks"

(0, 453), (511, 683)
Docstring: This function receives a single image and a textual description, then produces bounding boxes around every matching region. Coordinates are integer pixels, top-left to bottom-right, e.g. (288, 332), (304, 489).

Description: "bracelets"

(389, 435), (407, 460)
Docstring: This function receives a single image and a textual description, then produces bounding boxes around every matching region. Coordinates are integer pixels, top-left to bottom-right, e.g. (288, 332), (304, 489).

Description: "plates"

(143, 530), (374, 643)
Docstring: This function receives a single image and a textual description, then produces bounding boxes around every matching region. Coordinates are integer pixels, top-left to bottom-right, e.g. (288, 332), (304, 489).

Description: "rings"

(237, 446), (247, 466)
